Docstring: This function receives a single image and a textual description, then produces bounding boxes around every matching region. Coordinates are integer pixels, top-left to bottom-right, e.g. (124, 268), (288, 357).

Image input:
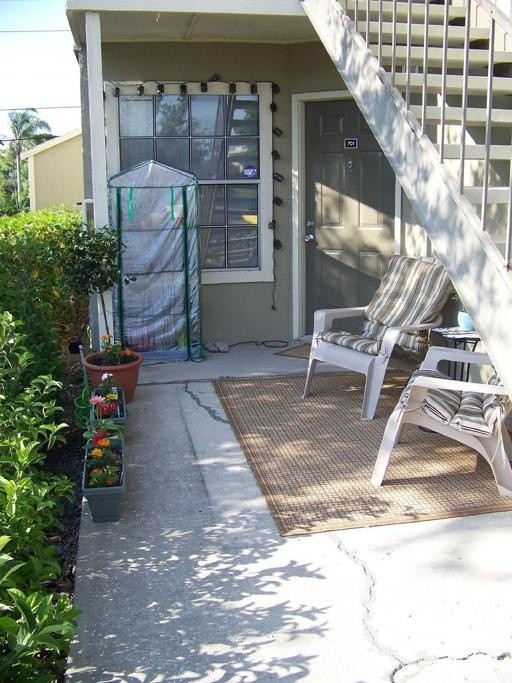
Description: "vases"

(83, 351), (143, 405)
(457, 310), (473, 332)
(82, 387), (128, 524)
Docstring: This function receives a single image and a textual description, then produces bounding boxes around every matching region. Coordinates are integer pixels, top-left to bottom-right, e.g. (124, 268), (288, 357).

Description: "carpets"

(214, 368), (512, 538)
(273, 341), (320, 360)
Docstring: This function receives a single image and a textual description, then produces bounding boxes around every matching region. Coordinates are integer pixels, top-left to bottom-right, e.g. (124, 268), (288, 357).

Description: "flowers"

(85, 372), (122, 487)
(98, 332), (132, 365)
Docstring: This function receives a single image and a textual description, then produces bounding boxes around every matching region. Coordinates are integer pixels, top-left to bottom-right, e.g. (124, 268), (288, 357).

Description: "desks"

(431, 327), (481, 383)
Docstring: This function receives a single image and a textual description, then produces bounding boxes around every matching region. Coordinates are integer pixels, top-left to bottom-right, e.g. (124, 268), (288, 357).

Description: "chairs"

(302, 255), (452, 420)
(371, 346), (512, 498)
